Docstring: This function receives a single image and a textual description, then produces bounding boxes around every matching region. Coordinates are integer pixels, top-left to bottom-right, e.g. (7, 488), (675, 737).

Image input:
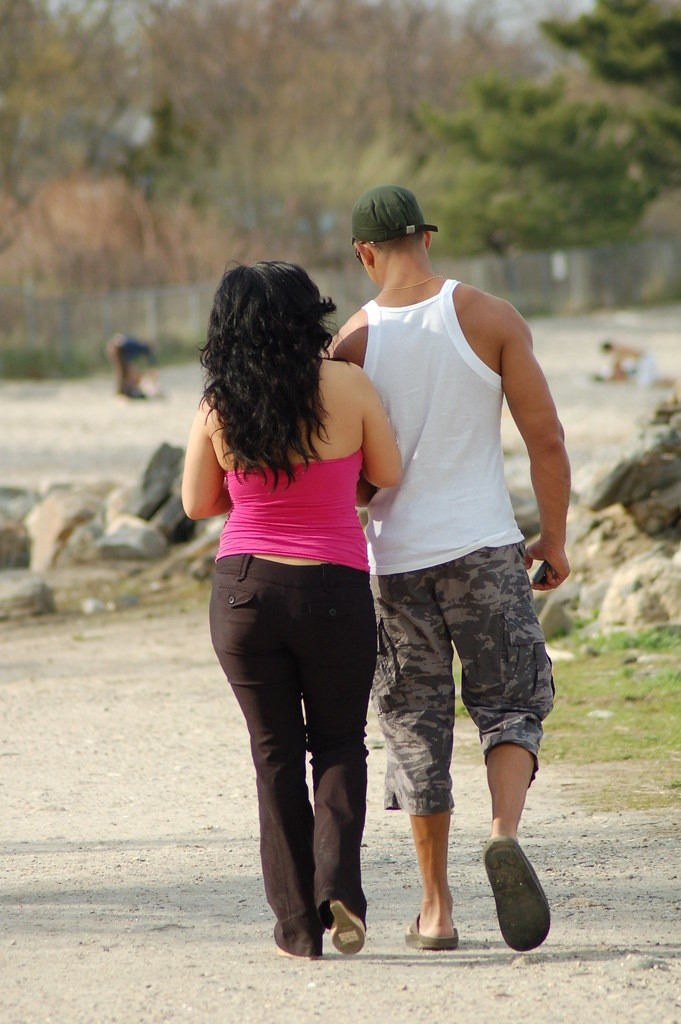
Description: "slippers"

(405, 913), (458, 949)
(482, 837), (550, 951)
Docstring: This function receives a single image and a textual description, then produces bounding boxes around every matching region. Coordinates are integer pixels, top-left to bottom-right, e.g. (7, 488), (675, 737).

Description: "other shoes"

(276, 946), (317, 960)
(330, 900), (366, 954)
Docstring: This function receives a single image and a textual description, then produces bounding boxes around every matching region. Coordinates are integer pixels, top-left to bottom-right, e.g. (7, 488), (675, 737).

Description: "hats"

(352, 184), (438, 245)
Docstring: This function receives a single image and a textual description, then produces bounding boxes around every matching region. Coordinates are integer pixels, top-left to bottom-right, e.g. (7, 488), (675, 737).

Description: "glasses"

(356, 240), (376, 265)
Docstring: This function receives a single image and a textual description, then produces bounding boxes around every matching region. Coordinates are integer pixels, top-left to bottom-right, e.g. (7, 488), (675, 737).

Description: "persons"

(105, 333), (160, 400)
(320, 183), (570, 950)
(181, 259), (401, 960)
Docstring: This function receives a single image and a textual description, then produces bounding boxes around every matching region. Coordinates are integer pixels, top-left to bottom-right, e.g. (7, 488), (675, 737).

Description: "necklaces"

(383, 276), (444, 291)
